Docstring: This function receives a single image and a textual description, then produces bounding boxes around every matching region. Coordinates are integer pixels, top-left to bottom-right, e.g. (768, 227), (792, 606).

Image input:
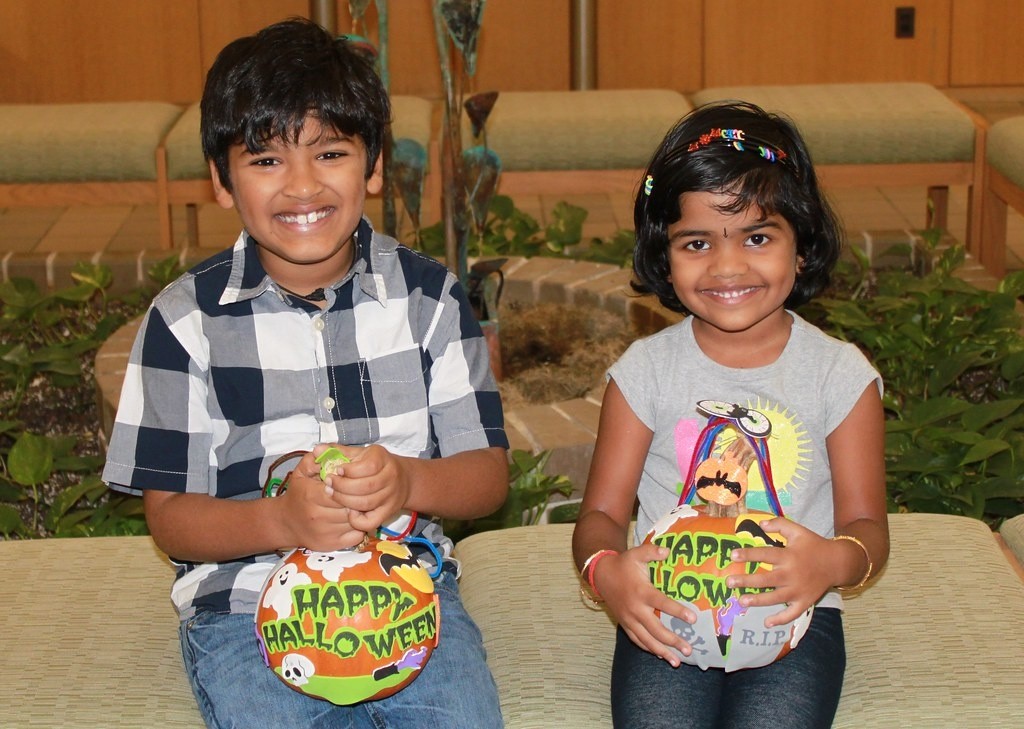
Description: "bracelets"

(578, 550), (621, 612)
(830, 535), (872, 591)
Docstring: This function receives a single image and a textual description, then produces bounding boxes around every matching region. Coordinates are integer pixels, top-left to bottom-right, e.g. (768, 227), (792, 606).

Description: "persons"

(574, 99), (889, 729)
(100, 17), (510, 729)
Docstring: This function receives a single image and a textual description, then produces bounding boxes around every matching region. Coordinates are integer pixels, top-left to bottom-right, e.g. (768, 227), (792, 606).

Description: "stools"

(1, 83), (1023, 299)
(0, 509), (1023, 728)
